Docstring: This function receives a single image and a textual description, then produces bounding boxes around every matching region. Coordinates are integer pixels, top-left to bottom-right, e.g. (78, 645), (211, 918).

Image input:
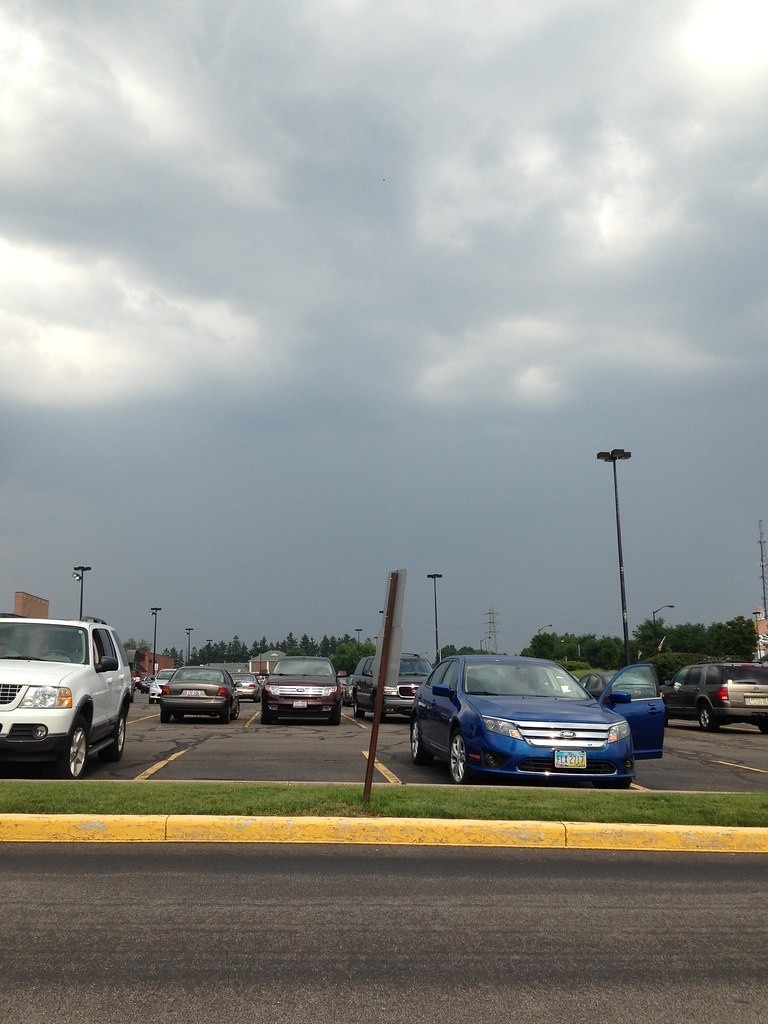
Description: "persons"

(133, 675), (142, 690)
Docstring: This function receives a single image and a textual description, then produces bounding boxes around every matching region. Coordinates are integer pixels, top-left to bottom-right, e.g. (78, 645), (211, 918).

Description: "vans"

(351, 653), (434, 719)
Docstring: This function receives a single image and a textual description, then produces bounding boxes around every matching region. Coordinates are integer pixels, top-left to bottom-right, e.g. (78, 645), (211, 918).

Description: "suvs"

(0, 611), (135, 782)
(658, 661), (768, 735)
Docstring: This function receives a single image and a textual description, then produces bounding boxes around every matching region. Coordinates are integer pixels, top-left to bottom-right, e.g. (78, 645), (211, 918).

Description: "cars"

(148, 668), (177, 704)
(159, 666), (242, 724)
(259, 656), (347, 726)
(141, 675), (156, 694)
(577, 673), (655, 702)
(410, 656), (666, 791)
(230, 672), (262, 703)
(336, 674), (356, 707)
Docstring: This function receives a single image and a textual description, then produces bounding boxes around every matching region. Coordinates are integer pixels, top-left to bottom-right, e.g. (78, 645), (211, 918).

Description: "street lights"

(653, 605), (675, 626)
(184, 628), (194, 663)
(538, 624), (552, 636)
(149, 607), (162, 676)
(355, 628), (362, 644)
(480, 637), (492, 654)
(72, 566), (91, 620)
(206, 640), (213, 666)
(597, 449), (631, 667)
(427, 574), (443, 665)
(752, 611), (762, 661)
